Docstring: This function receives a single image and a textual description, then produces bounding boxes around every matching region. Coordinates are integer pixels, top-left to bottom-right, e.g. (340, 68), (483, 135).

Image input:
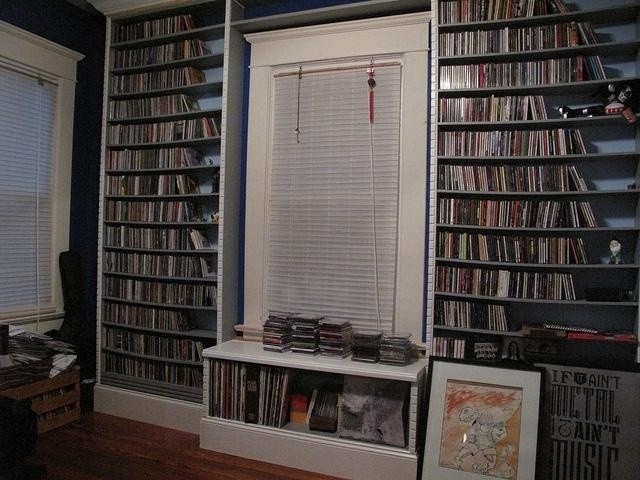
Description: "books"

(106, 328), (206, 388)
(209, 360), (292, 429)
(436, 232), (589, 301)
(433, 300), (514, 359)
(109, 65), (207, 119)
(439, 97), (586, 157)
(105, 225), (216, 277)
(0, 332), (79, 391)
(104, 276), (217, 331)
(114, 14), (211, 67)
(439, 1), (577, 23)
(439, 166), (600, 228)
(262, 311), (413, 366)
(439, 23), (605, 89)
(105, 172), (203, 221)
(105, 116), (221, 170)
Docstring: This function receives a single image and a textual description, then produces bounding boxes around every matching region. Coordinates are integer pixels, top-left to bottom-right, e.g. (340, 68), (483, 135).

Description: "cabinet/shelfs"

(421, 0), (640, 479)
(199, 338), (428, 480)
(93, 1), (246, 434)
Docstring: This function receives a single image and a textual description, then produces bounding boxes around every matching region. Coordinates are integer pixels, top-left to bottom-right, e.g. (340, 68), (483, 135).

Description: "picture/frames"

(420, 355), (542, 480)
